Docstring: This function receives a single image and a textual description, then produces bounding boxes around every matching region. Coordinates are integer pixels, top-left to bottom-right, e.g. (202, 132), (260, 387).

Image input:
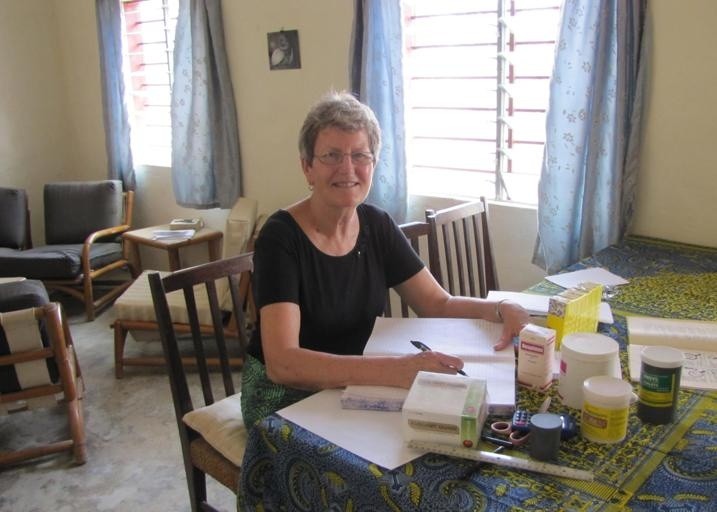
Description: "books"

(340, 315), (517, 418)
(625, 315), (717, 391)
(486, 290), (614, 333)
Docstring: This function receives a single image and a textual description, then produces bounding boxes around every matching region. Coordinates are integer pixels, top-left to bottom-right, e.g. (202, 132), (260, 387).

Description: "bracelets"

(496, 298), (514, 323)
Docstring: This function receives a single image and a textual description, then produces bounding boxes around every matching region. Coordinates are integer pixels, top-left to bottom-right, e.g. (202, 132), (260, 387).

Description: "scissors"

(490, 421), (535, 446)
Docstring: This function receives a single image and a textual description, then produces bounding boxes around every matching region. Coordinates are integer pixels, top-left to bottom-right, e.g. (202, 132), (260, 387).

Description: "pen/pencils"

(481, 433), (512, 446)
(409, 340), (470, 379)
(457, 445), (505, 481)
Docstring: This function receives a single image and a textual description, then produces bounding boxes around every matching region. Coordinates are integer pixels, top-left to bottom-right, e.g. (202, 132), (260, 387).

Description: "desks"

(236, 234), (716, 511)
(121, 223), (223, 273)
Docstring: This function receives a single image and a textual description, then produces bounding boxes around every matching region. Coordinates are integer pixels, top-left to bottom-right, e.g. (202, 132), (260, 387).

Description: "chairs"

(145, 252), (249, 512)
(382, 213), (442, 318)
(424, 195), (500, 301)
(0, 186), (33, 256)
(0, 178), (139, 323)
(1, 278), (89, 468)
(107, 195), (270, 379)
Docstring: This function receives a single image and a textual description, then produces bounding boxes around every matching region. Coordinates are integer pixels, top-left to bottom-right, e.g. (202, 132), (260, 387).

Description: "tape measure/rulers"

(408, 440), (595, 484)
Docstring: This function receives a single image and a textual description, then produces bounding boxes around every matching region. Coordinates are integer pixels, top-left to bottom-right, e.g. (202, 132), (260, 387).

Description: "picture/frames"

(265, 28), (302, 72)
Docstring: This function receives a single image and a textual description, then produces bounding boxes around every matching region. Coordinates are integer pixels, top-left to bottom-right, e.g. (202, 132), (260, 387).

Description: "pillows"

(180, 389), (254, 470)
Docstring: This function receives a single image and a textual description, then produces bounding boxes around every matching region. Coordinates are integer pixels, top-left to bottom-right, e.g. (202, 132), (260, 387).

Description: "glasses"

(313, 150), (375, 166)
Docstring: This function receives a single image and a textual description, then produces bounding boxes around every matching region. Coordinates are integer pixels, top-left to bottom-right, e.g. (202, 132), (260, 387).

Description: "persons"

(241, 91), (531, 436)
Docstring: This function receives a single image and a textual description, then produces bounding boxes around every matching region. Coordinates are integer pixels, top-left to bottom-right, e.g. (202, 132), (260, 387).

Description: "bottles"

(579, 376), (631, 446)
(636, 342), (683, 420)
(555, 332), (620, 407)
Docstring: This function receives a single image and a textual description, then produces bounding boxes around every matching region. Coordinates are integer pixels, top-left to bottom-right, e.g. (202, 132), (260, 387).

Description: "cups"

(526, 412), (563, 459)
